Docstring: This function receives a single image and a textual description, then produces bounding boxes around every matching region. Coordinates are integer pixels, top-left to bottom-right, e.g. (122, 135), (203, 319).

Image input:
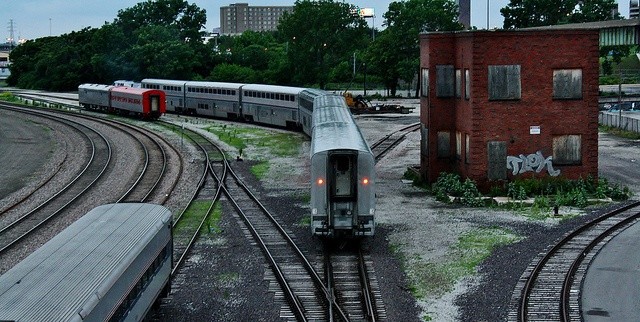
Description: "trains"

(78, 84), (166, 120)
(0, 203), (172, 322)
(141, 79), (375, 238)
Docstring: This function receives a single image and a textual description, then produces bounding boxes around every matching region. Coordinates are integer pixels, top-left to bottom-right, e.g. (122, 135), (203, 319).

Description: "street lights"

(321, 44), (326, 87)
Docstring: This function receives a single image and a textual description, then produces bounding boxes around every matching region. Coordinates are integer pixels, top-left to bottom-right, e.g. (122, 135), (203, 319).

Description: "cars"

(609, 103), (638, 110)
